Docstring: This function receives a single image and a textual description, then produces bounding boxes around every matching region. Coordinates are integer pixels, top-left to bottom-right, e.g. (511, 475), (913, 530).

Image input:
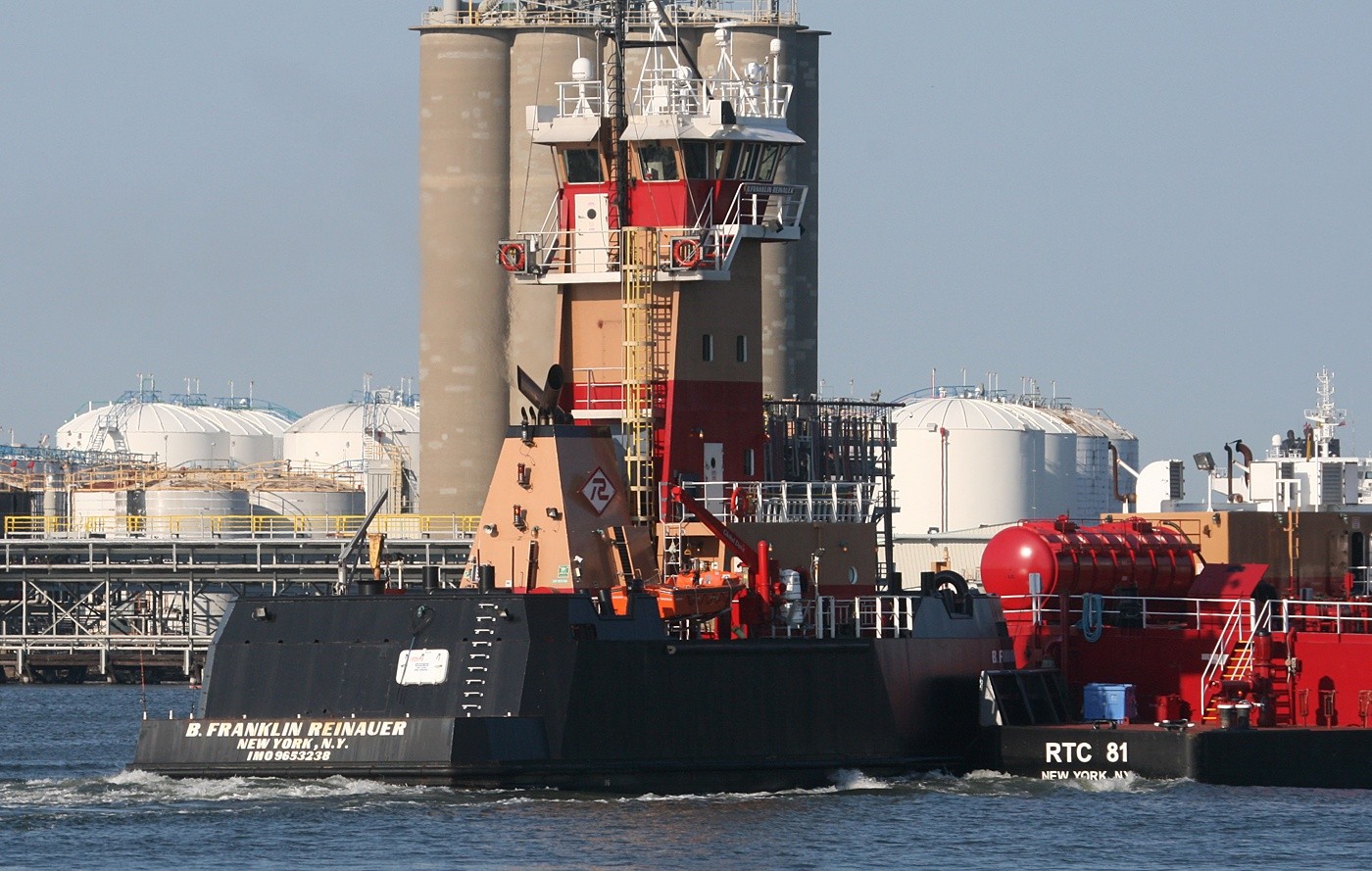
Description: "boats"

(949, 371), (1372, 794)
(136, 0), (1014, 794)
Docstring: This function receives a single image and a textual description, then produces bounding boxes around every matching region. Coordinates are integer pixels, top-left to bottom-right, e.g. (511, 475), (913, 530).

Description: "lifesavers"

(671, 238), (701, 269)
(729, 487), (749, 519)
(499, 243), (525, 270)
